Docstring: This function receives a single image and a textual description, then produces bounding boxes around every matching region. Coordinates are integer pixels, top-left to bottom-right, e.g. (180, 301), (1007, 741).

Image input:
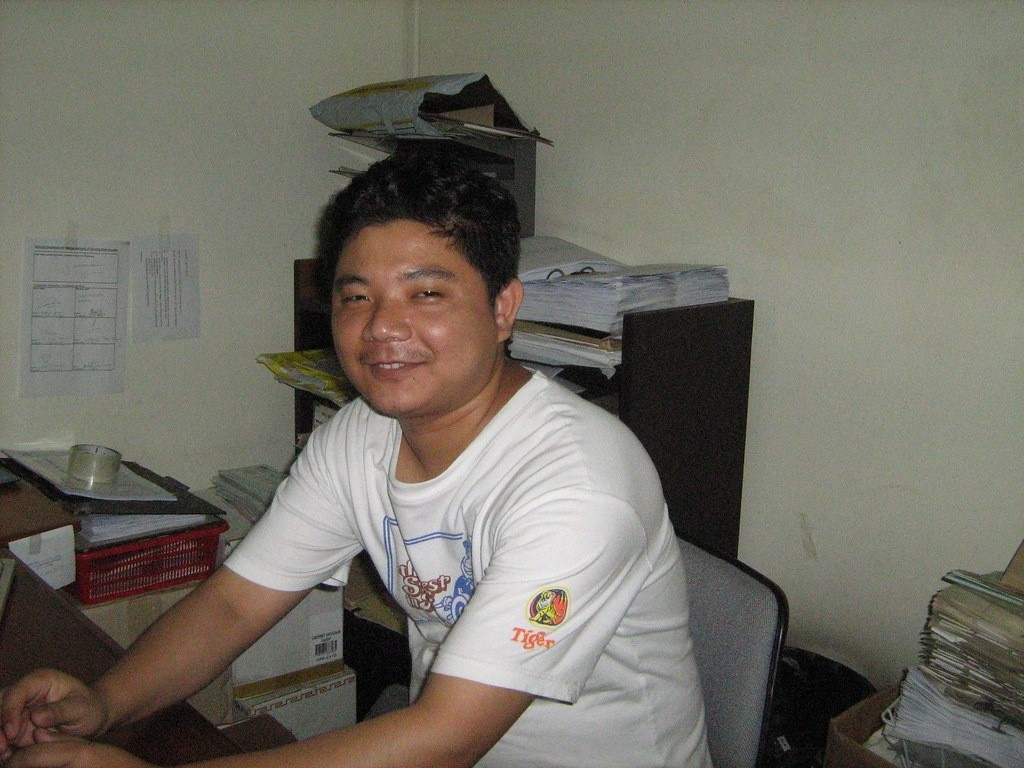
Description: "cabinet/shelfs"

(292, 253), (754, 722)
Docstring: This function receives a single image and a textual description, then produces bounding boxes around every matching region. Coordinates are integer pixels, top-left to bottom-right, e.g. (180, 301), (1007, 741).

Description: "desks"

(0, 549), (248, 768)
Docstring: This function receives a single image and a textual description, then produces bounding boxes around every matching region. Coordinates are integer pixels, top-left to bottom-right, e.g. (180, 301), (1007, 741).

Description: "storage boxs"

(233, 670), (359, 743)
(195, 487), (344, 700)
(72, 517), (229, 605)
(78, 607), (234, 728)
(828, 688), (913, 768)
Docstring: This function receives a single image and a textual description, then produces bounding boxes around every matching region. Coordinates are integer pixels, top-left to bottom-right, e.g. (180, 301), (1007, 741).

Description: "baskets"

(68, 516), (231, 609)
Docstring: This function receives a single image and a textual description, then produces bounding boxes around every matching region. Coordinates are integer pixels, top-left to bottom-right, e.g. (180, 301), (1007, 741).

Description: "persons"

(0, 147), (713, 768)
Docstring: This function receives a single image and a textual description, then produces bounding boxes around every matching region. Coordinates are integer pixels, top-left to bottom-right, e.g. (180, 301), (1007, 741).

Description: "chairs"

(676, 534), (789, 768)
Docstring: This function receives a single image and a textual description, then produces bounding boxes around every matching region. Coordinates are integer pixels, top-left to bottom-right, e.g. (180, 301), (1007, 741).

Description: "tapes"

(67, 443), (123, 487)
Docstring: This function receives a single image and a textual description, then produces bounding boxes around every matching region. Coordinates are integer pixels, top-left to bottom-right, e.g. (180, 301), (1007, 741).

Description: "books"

(210, 238), (1024, 768)
